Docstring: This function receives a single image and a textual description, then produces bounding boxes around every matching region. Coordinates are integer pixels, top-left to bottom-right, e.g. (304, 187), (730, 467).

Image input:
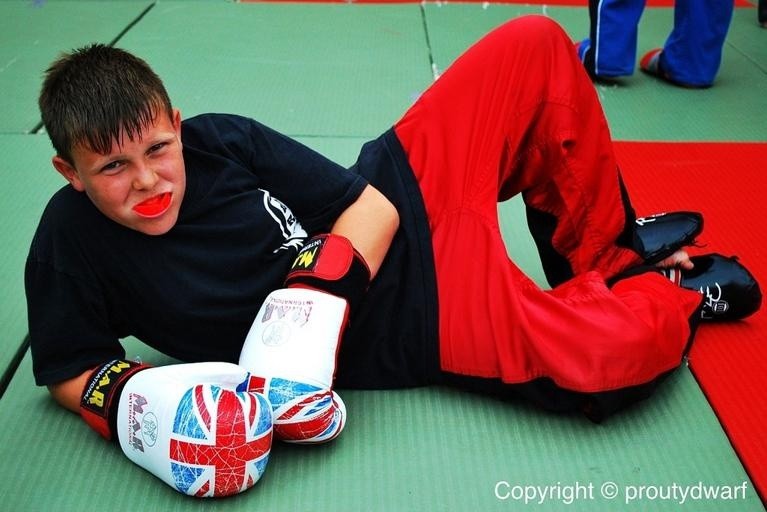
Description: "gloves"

(235, 231), (372, 447)
(78, 357), (275, 500)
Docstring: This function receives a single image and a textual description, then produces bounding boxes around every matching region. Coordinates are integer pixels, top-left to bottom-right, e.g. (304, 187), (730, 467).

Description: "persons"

(565, 0), (743, 89)
(24, 14), (762, 501)
